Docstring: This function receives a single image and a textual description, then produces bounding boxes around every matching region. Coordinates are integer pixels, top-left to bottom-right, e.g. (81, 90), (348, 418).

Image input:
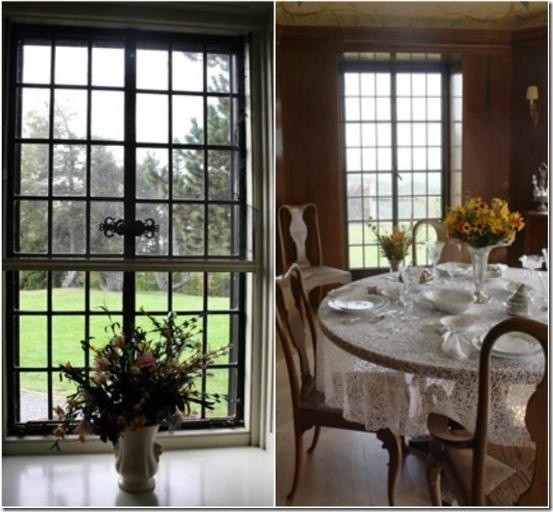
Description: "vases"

(114, 424), (161, 493)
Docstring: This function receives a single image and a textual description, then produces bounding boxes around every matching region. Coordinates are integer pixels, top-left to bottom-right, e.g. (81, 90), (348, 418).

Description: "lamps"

(525, 84), (540, 125)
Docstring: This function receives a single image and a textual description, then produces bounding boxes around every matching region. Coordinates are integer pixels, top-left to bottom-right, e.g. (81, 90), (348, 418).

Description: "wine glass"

(424, 238), (446, 282)
(398, 265), (421, 323)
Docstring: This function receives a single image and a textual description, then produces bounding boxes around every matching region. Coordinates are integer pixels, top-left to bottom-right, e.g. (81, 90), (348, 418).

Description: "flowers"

(48, 305), (241, 446)
(440, 190), (526, 248)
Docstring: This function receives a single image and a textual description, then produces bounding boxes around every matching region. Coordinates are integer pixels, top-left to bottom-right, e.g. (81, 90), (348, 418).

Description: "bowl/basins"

(439, 314), (478, 333)
(420, 286), (480, 313)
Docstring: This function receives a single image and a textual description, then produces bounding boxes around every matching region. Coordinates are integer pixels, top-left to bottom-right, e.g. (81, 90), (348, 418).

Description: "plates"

(327, 292), (391, 315)
(435, 261), (474, 276)
(471, 330), (545, 361)
